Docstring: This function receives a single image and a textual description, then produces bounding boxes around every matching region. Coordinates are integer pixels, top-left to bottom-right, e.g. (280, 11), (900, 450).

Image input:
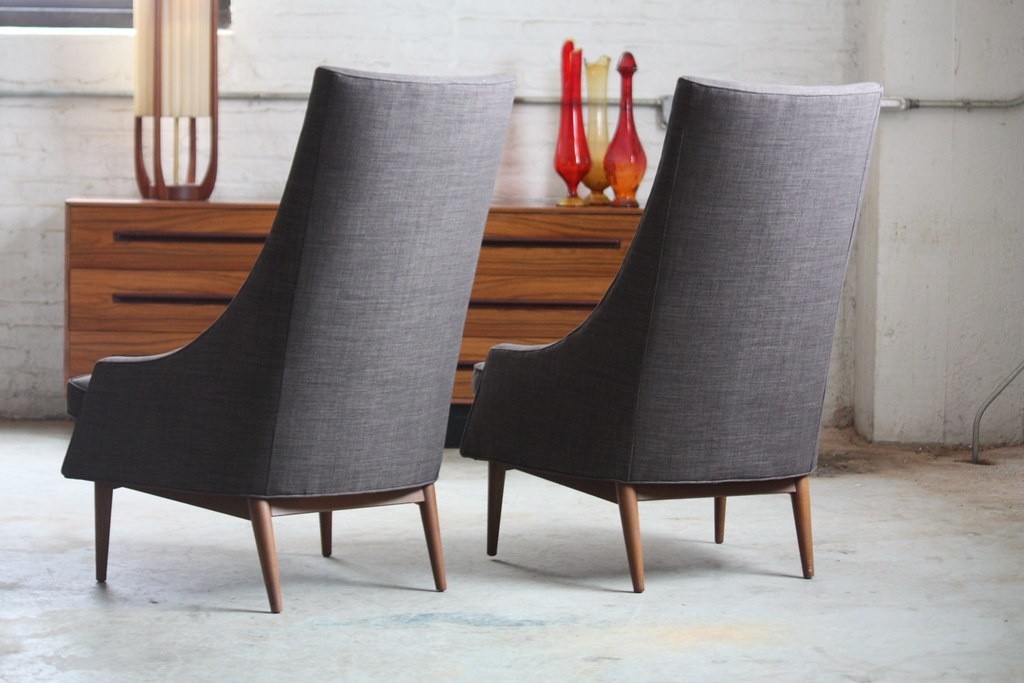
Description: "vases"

(580, 54), (613, 207)
(601, 50), (647, 208)
(552, 40), (591, 208)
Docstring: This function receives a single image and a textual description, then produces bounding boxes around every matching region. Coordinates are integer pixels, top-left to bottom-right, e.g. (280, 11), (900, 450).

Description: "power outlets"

(657, 93), (673, 129)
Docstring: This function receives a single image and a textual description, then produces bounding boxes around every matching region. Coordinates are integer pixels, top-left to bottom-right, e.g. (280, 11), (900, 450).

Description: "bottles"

(555, 40), (647, 209)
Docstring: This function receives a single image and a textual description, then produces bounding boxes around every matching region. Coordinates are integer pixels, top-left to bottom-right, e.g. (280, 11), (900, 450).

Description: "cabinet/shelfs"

(59, 198), (644, 449)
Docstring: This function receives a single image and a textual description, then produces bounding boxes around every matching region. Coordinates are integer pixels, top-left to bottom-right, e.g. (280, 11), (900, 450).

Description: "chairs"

(60, 66), (519, 614)
(459, 75), (886, 595)
